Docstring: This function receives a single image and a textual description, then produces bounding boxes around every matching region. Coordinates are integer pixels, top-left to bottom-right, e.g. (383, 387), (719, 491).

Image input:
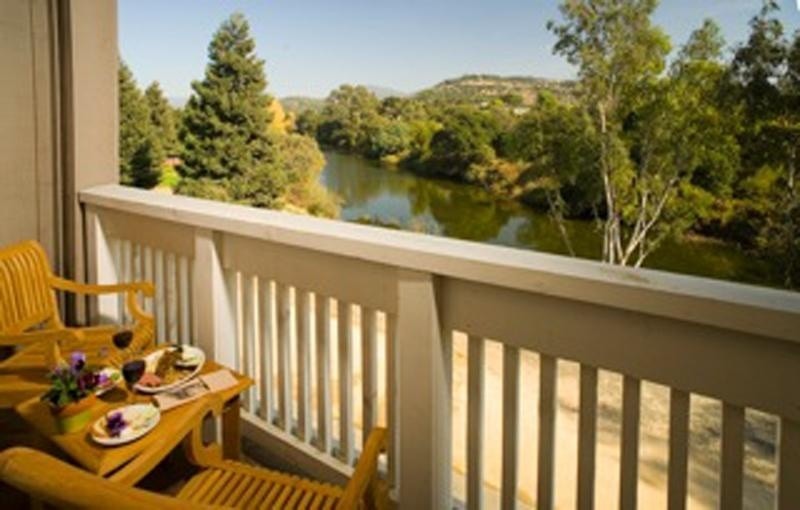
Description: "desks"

(15, 342), (254, 480)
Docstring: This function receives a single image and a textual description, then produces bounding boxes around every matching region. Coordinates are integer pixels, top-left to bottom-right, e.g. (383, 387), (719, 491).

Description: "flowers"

(40, 351), (111, 407)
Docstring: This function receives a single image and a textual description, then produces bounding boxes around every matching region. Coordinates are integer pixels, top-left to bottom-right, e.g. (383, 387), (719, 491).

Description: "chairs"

(0, 391), (392, 510)
(0, 240), (157, 417)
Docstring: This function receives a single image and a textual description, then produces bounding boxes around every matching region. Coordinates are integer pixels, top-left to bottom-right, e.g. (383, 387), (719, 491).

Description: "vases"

(49, 392), (96, 435)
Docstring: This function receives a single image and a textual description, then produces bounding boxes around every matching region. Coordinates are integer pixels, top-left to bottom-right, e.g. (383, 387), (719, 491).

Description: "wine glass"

(109, 321), (133, 367)
(121, 357), (147, 406)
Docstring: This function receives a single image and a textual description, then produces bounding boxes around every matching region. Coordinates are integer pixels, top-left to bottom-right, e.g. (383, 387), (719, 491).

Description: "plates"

(131, 343), (206, 394)
(91, 403), (161, 446)
(87, 364), (121, 398)
